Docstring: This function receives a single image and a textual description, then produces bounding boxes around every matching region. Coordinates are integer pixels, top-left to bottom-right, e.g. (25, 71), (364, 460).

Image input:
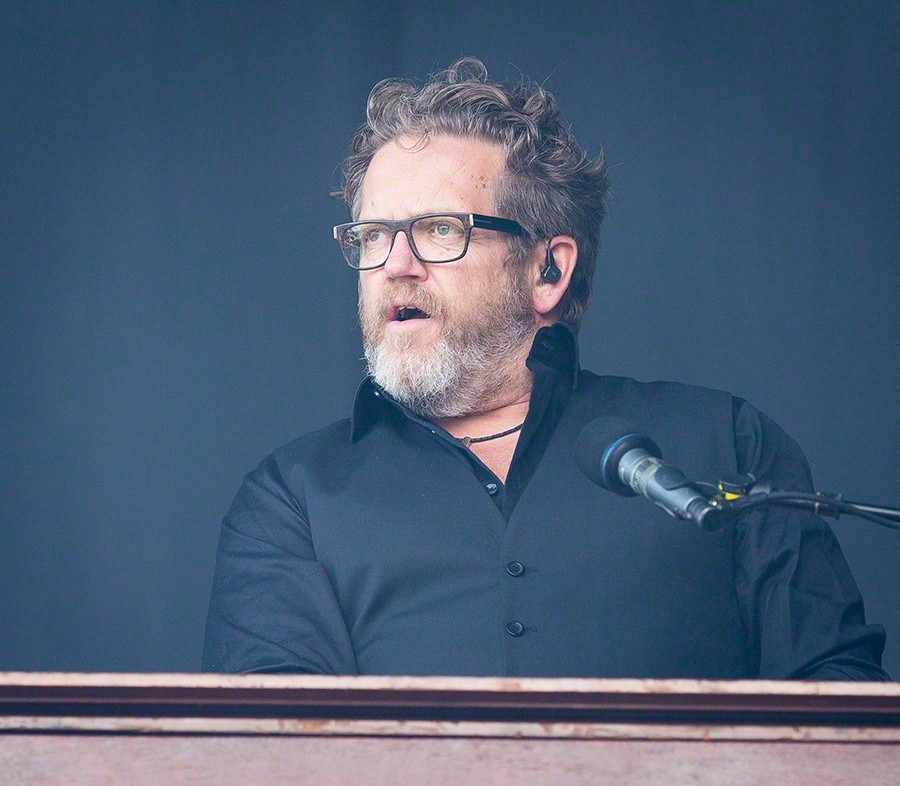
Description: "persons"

(201, 58), (895, 716)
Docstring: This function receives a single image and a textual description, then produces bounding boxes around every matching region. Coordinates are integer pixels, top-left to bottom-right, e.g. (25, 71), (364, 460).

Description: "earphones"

(540, 250), (562, 283)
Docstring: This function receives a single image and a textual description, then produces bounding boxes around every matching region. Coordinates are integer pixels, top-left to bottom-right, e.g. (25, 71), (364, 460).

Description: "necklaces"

(455, 425), (523, 448)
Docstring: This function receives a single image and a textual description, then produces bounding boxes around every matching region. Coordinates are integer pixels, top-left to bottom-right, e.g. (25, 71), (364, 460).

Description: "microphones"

(575, 414), (725, 533)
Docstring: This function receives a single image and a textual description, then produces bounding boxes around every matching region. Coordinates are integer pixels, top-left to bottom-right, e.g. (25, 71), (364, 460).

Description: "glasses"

(333, 213), (529, 270)
